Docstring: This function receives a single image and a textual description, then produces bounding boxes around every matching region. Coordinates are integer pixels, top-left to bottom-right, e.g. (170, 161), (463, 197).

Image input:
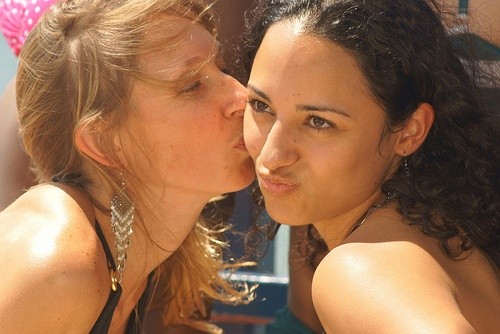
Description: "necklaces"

(345, 192), (394, 237)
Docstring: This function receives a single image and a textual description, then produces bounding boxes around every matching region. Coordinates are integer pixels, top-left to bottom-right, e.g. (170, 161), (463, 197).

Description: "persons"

(0, 0), (257, 334)
(242, 0), (499, 334)
